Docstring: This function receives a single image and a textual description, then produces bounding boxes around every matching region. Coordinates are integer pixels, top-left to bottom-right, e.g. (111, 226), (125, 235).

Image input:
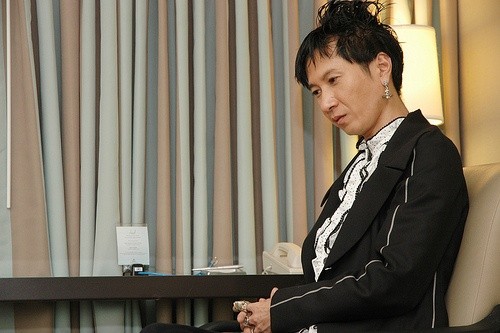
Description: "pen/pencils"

(138, 272), (175, 276)
(192, 266), (243, 271)
(201, 256), (221, 276)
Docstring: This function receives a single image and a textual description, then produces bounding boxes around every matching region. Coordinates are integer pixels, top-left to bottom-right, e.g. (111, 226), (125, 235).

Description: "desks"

(1, 274), (304, 302)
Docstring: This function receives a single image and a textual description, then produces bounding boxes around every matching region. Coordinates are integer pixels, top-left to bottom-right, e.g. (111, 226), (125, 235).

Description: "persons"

(139, 1), (470, 333)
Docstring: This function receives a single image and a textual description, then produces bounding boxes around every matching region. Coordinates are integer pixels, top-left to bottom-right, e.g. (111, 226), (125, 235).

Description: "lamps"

(388, 24), (443, 126)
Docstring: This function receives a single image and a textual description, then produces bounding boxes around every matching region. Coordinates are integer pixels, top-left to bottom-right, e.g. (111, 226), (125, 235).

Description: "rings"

(249, 328), (256, 333)
(242, 317), (250, 327)
(232, 300), (250, 313)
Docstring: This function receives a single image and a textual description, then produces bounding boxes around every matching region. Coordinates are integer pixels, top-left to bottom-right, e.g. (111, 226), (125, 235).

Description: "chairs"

(199, 163), (500, 333)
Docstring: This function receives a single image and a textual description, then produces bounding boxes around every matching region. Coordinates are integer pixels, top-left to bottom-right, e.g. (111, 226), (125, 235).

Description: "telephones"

(263, 243), (303, 274)
